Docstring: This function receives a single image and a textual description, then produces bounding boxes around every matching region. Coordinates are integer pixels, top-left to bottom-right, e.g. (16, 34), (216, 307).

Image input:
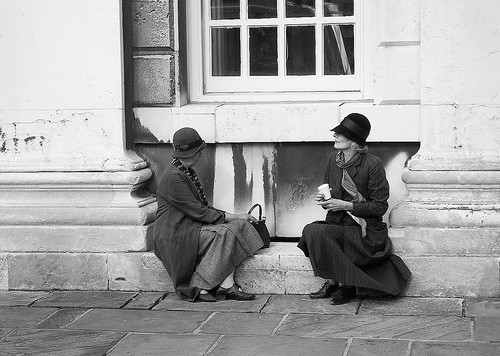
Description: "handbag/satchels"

(248, 203), (271, 249)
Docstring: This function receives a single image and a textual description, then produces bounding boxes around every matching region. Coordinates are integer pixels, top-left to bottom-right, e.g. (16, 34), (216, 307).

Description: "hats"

(172, 127), (206, 158)
(329, 113), (371, 147)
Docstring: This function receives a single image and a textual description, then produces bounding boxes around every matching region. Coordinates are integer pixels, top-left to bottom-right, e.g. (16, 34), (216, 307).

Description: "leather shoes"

(331, 284), (357, 305)
(309, 281), (339, 298)
(216, 285), (255, 301)
(197, 290), (217, 302)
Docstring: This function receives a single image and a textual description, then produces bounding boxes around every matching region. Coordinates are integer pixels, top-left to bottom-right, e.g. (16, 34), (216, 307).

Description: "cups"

(317, 184), (331, 200)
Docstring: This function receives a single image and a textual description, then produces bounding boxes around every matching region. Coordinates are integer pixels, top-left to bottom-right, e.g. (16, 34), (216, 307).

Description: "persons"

(296, 113), (411, 306)
(152, 127), (265, 303)
